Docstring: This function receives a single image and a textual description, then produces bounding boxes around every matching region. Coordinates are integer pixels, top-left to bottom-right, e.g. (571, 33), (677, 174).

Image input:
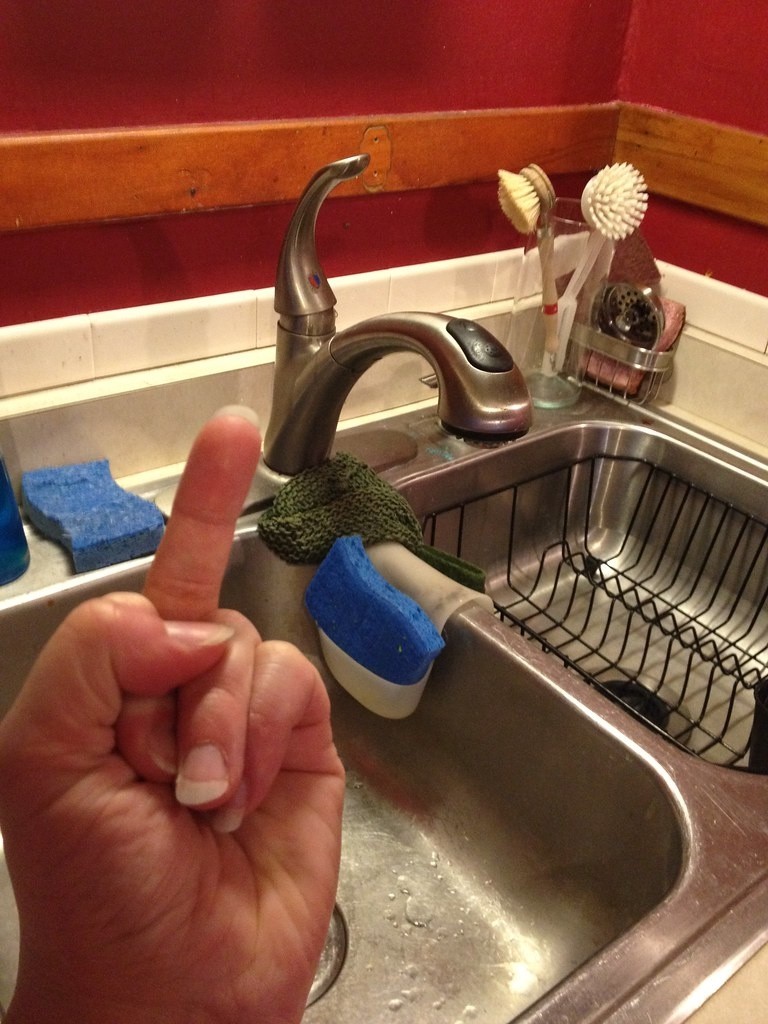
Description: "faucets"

(260, 152), (537, 479)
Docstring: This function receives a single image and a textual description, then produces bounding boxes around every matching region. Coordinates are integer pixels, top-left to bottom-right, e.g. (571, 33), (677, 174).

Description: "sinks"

(0, 517), (689, 1024)
(382, 421), (768, 782)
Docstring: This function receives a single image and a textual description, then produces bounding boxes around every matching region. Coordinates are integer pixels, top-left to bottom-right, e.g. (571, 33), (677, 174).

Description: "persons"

(0, 408), (344, 1024)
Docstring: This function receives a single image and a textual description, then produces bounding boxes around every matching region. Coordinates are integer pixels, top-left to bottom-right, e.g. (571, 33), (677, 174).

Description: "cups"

(504, 198), (609, 411)
